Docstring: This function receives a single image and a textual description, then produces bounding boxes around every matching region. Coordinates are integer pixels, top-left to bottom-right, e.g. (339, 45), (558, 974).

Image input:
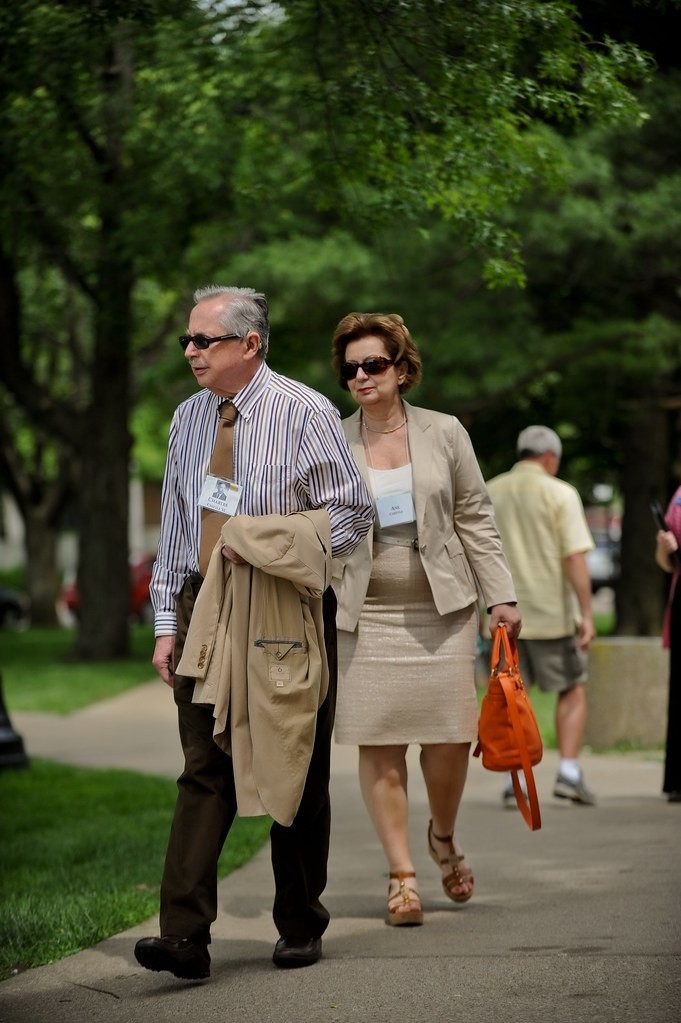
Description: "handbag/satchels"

(473, 624), (542, 832)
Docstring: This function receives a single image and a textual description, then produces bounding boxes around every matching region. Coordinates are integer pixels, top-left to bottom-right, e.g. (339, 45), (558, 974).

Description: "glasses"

(342, 357), (396, 380)
(179, 332), (239, 350)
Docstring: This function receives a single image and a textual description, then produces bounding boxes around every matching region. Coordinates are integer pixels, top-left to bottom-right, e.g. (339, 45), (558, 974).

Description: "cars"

(56, 553), (159, 661)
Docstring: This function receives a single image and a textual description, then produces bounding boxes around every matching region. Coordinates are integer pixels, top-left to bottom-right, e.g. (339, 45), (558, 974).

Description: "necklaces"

(365, 420), (408, 434)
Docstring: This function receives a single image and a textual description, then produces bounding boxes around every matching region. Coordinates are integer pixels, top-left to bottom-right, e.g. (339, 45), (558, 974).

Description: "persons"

(212, 482), (227, 501)
(655, 485), (681, 803)
(332, 313), (523, 926)
(477, 424), (598, 807)
(134, 284), (374, 980)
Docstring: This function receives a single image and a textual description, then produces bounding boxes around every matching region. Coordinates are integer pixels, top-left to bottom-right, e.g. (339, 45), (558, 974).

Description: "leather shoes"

(135, 936), (210, 980)
(270, 935), (322, 965)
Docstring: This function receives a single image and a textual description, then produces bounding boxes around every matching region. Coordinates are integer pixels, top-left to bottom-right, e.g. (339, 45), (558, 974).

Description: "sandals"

(427, 819), (474, 902)
(388, 871), (423, 924)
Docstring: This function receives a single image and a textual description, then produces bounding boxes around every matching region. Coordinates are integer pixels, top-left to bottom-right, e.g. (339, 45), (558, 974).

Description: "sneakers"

(503, 784), (528, 809)
(554, 770), (594, 805)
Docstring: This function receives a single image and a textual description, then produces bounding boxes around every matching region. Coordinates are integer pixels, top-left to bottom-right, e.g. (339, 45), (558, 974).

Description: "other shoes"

(667, 792), (681, 802)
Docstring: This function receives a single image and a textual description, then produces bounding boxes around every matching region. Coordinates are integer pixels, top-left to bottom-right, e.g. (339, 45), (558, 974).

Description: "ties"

(199, 403), (238, 580)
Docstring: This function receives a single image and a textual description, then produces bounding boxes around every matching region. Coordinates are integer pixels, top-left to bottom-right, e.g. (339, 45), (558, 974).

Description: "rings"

(517, 627), (521, 632)
